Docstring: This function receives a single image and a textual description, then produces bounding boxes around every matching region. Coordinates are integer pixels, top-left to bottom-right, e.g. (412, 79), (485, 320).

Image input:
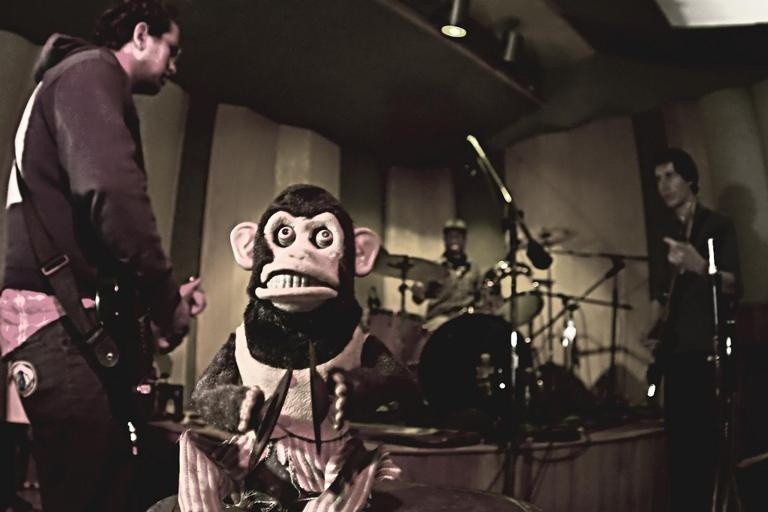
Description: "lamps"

(441, 0), (470, 38)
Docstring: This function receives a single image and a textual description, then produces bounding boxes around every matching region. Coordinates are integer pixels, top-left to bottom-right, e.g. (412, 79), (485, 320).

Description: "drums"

(364, 312), (424, 365)
(479, 258), (543, 328)
(418, 310), (540, 428)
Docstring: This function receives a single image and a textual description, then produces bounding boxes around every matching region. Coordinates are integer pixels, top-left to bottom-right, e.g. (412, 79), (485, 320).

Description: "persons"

(410, 214), (493, 335)
(1, 1), (207, 509)
(638, 144), (742, 511)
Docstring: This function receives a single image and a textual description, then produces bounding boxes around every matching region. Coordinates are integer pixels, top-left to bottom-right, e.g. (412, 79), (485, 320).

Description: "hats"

(443, 218), (467, 234)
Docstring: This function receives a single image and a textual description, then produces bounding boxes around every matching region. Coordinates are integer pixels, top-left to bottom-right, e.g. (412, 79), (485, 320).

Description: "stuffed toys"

(177, 180), (420, 511)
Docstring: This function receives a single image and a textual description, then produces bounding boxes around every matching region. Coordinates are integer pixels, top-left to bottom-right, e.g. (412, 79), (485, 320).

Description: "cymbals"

(372, 253), (451, 282)
(517, 228), (571, 252)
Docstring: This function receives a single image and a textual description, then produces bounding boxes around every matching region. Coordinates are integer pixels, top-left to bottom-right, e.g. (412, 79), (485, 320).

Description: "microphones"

(463, 141), (476, 177)
